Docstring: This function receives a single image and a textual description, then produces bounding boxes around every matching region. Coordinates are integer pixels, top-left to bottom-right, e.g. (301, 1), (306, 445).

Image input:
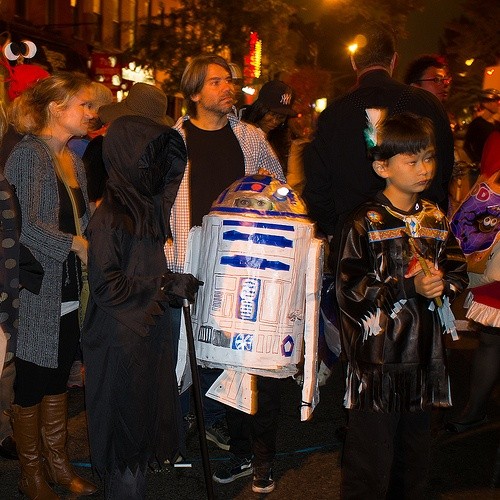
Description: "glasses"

(418, 75), (455, 87)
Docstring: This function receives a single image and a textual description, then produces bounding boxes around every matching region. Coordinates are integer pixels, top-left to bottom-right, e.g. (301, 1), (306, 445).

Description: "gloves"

(158, 272), (204, 307)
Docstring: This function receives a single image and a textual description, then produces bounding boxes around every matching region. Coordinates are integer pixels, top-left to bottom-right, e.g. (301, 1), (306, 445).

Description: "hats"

(257, 80), (298, 115)
(99, 83), (175, 130)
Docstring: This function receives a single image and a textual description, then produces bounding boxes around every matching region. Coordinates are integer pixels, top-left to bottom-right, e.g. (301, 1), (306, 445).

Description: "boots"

(39, 392), (98, 495)
(10, 403), (62, 500)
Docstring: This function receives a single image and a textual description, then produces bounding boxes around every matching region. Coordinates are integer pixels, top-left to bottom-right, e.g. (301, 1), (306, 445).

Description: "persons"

(290, 49), (331, 101)
(131, 23), (170, 61)
(0, 52), (313, 500)
(301, 21), (500, 500)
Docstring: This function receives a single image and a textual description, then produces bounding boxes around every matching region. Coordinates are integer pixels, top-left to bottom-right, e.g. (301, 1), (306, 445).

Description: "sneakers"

(212, 454), (254, 484)
(251, 462), (275, 492)
